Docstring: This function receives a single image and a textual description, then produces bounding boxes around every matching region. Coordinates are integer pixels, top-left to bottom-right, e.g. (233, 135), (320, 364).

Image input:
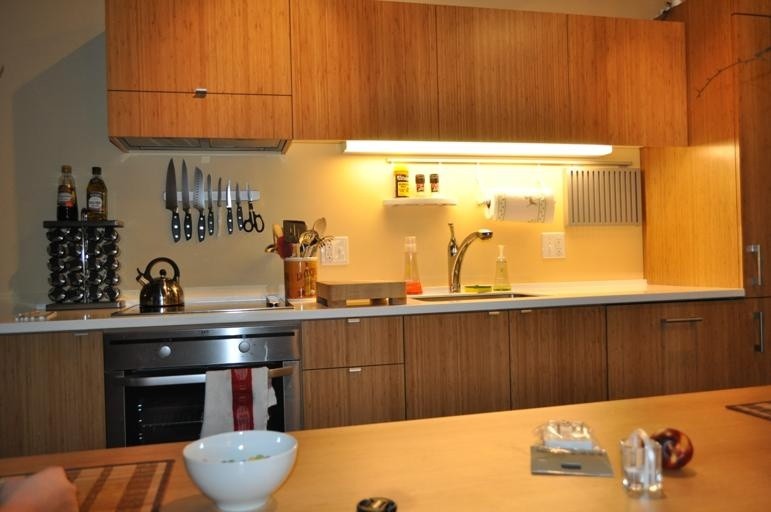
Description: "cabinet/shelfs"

(639, 2), (771, 296)
(288, 0), (690, 151)
(606, 297), (770, 399)
(104, 321), (302, 451)
(301, 314), (404, 433)
(404, 304), (607, 423)
(4, 331), (107, 453)
(102, 1), (292, 154)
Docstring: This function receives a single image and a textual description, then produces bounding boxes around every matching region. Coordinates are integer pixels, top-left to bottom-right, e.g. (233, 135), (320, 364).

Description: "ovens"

(105, 358), (303, 450)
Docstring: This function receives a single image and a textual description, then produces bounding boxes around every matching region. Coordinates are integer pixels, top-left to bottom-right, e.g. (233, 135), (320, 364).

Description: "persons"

(0, 464), (78, 512)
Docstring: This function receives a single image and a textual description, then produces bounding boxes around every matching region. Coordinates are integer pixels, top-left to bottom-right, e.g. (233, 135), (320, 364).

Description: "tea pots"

(136, 257), (184, 307)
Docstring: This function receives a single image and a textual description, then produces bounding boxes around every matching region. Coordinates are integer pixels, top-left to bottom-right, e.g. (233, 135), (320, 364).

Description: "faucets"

(447, 222), (494, 293)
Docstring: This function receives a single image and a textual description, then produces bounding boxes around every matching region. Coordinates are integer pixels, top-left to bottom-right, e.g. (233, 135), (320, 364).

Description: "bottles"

(87, 166), (108, 222)
(57, 165), (79, 220)
(430, 173), (441, 192)
(395, 167), (411, 198)
(416, 174), (425, 192)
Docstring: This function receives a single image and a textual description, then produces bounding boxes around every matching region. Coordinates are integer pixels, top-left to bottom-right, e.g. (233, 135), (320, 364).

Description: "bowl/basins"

(182, 428), (299, 511)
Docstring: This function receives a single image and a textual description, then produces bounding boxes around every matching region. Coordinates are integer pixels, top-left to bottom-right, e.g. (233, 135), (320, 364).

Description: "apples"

(642, 428), (693, 470)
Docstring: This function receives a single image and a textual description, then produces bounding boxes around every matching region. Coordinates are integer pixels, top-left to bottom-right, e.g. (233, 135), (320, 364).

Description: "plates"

(110, 292), (292, 316)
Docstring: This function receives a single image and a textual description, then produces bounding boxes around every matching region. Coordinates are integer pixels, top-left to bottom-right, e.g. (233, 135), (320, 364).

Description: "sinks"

(407, 292), (554, 302)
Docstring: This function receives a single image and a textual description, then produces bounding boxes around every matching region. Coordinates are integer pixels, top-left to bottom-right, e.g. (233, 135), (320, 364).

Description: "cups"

(286, 257), (320, 304)
(622, 438), (665, 488)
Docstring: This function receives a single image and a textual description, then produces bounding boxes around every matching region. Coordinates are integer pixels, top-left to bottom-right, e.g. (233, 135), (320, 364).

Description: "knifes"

(207, 174), (214, 233)
(182, 159), (192, 240)
(167, 158), (181, 242)
(236, 181), (244, 228)
(225, 178), (234, 232)
(193, 166), (206, 242)
(217, 177), (223, 237)
